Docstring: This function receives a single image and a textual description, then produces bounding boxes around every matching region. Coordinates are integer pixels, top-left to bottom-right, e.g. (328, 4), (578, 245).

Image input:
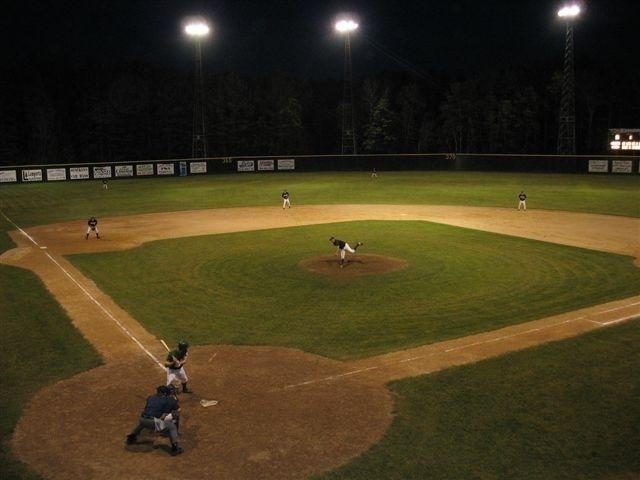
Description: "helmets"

(156, 385), (170, 396)
(178, 341), (190, 352)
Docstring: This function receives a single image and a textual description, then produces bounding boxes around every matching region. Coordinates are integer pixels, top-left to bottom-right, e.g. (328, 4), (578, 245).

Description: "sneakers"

(170, 441), (184, 456)
(122, 432), (138, 446)
(183, 389), (193, 393)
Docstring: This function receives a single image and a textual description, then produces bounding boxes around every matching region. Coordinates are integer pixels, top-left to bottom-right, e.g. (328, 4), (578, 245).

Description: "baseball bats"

(160, 339), (177, 361)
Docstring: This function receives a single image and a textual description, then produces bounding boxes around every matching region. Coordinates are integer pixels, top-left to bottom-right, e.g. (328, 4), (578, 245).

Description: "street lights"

(557, 2), (585, 154)
(336, 16), (362, 155)
(182, 16), (212, 157)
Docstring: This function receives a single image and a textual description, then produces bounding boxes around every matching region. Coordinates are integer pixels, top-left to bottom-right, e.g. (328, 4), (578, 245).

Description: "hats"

(329, 236), (334, 241)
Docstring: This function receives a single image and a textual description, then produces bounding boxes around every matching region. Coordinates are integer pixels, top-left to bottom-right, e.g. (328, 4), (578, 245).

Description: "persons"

(328, 236), (364, 269)
(280, 188), (292, 210)
(165, 339), (195, 394)
(371, 167), (378, 179)
(102, 177), (109, 190)
(124, 383), (184, 456)
(517, 190), (527, 211)
(85, 215), (100, 241)
